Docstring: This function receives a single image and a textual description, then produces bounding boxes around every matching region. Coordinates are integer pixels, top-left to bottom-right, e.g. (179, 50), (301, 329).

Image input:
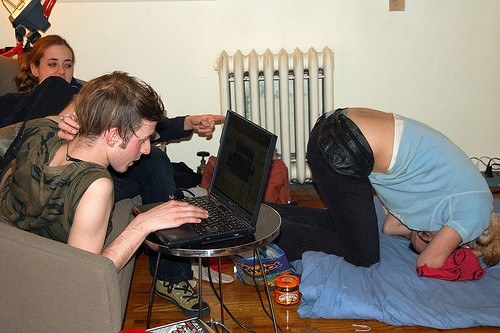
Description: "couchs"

(0, 55), (146, 333)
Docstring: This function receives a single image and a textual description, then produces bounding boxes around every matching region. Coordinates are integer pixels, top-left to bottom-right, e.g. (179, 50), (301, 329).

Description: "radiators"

(213, 45), (337, 186)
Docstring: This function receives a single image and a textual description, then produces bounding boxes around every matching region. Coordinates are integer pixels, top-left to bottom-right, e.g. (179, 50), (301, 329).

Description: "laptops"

(132, 109), (279, 248)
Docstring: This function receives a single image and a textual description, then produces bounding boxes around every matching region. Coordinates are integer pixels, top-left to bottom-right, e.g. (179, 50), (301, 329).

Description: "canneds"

(274, 275), (300, 306)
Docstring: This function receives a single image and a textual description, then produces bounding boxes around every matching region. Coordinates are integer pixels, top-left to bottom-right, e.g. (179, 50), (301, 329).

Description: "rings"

(60, 114), (72, 121)
(209, 120), (215, 126)
(200, 120), (204, 123)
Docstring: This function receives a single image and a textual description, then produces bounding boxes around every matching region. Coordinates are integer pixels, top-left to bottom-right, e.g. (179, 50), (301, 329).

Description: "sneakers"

(154, 279), (210, 317)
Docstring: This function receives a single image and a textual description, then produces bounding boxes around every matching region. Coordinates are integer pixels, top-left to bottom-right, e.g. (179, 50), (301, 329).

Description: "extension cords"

(480, 171), (500, 186)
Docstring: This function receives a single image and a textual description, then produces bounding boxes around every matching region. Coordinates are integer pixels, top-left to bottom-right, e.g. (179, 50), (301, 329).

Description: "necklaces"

(66, 141), (85, 163)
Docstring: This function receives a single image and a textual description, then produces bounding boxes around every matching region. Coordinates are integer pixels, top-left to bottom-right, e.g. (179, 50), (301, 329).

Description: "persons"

(0, 35), (226, 317)
(262, 108), (493, 268)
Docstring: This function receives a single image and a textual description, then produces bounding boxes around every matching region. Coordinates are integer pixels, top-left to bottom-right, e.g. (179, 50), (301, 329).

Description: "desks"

(129, 202), (282, 333)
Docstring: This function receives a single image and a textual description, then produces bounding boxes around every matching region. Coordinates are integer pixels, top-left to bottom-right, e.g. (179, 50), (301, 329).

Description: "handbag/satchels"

(200, 156), (291, 204)
